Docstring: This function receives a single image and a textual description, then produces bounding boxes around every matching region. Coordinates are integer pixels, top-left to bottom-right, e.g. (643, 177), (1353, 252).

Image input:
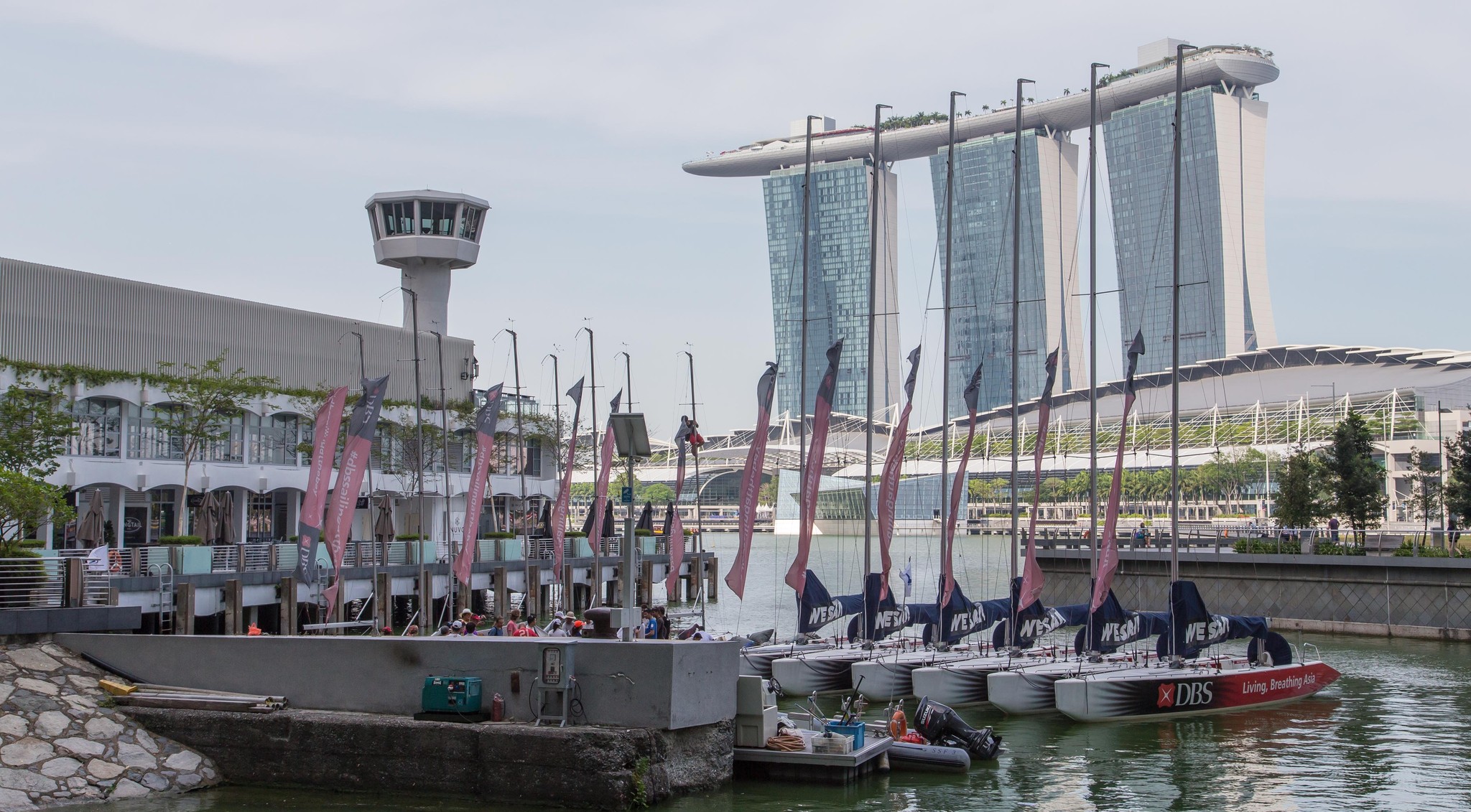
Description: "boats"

(777, 712), (974, 775)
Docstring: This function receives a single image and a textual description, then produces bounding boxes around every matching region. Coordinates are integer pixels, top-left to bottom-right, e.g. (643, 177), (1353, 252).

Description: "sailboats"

(729, 43), (1342, 726)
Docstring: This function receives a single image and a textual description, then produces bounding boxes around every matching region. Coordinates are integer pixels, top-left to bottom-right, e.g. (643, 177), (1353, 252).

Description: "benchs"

(1021, 528), (1248, 550)
(1357, 535), (1404, 556)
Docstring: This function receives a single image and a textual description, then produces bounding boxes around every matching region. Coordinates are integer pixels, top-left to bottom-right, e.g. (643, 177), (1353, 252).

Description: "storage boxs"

(823, 721), (865, 750)
(811, 733), (855, 754)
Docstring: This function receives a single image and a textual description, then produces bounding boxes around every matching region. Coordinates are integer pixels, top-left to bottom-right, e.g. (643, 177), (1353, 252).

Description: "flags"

(551, 376), (584, 583)
(586, 387), (624, 559)
(299, 384), (350, 587)
(939, 362), (983, 609)
(722, 362), (779, 601)
(1091, 330), (1145, 621)
(664, 416), (692, 598)
(453, 383), (505, 590)
(785, 337), (845, 599)
(322, 372), (392, 617)
(876, 345), (925, 598)
(1016, 346), (1062, 610)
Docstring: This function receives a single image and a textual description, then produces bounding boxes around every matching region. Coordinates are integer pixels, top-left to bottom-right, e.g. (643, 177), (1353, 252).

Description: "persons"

(1139, 522), (1154, 548)
(1447, 520), (1464, 558)
(1248, 520), (1297, 542)
(377, 603), (718, 643)
(680, 415), (705, 457)
(1327, 516), (1342, 547)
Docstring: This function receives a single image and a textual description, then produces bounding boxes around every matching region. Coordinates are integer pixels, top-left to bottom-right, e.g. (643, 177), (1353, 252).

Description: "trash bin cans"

(1430, 528), (1442, 547)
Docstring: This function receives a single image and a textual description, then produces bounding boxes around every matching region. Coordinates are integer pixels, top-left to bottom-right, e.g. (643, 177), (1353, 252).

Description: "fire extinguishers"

(493, 693), (506, 722)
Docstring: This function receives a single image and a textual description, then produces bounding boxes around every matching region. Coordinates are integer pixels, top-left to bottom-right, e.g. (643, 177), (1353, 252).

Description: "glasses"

(448, 622), (451, 626)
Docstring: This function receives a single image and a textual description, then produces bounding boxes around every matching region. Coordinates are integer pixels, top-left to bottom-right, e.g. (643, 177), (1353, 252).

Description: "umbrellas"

(75, 487), (105, 550)
(374, 494), (679, 566)
(196, 488), (235, 548)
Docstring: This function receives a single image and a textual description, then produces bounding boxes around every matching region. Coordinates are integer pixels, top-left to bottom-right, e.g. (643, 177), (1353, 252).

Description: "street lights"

(1437, 399), (1453, 550)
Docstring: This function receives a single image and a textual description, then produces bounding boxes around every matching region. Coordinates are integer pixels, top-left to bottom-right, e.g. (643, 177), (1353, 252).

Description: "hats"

(452, 620), (462, 628)
(574, 621), (586, 627)
(471, 614), (481, 619)
(564, 611), (576, 619)
(459, 608), (473, 618)
(554, 619), (561, 625)
(556, 611), (566, 619)
(382, 627), (391, 633)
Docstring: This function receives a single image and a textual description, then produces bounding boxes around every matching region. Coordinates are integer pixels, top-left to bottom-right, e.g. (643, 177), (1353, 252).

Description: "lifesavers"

(888, 710), (908, 740)
